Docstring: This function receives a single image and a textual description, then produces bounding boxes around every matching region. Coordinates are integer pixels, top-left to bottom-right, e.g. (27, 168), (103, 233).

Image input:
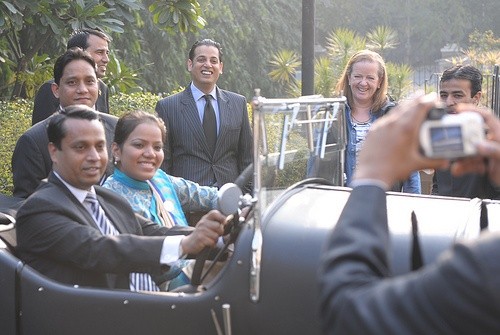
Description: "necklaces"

(350, 112), (371, 123)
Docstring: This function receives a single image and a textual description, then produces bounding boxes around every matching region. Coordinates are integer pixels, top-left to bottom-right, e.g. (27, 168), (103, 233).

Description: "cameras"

(418, 102), (486, 160)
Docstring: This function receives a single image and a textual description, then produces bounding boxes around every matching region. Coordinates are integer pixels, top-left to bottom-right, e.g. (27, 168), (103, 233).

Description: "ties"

(202, 94), (218, 160)
(85, 192), (161, 293)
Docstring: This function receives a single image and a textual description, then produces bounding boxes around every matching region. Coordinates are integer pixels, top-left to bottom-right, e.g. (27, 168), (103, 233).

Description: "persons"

(100, 110), (244, 294)
(153, 38), (256, 227)
(31, 30), (110, 126)
(309, 88), (500, 335)
(309, 49), (422, 195)
(9, 48), (121, 199)
(432, 65), (500, 200)
(14, 105), (230, 292)
